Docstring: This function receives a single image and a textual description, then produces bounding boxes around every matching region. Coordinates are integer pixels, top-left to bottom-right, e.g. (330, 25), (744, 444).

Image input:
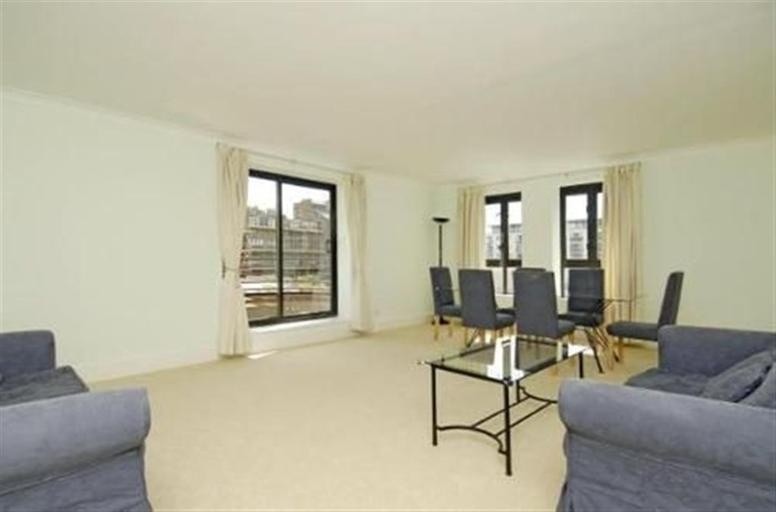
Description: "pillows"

(699, 349), (776, 410)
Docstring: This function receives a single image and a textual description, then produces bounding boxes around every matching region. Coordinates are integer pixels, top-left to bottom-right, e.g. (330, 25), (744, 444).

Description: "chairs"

(0, 329), (155, 512)
(557, 325), (776, 512)
(428, 262), (685, 376)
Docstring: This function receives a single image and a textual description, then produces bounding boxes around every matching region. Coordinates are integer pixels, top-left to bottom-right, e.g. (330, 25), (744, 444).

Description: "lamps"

(432, 216), (450, 325)
